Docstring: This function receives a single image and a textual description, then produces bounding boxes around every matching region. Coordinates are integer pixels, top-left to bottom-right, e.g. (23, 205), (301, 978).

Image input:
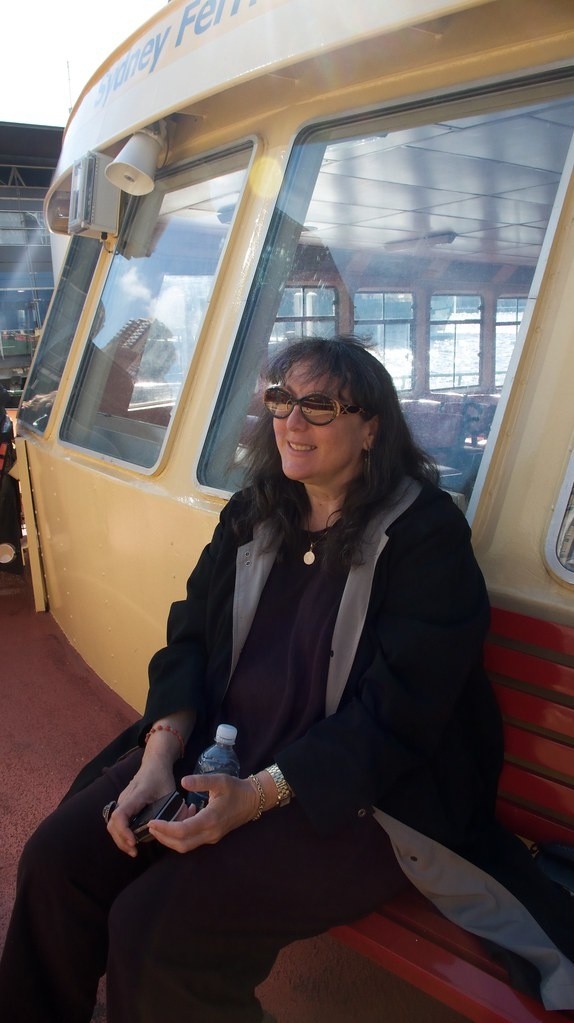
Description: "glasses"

(263, 386), (370, 425)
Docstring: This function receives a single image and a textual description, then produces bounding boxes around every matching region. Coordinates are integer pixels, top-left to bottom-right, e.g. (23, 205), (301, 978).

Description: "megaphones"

(105, 129), (164, 197)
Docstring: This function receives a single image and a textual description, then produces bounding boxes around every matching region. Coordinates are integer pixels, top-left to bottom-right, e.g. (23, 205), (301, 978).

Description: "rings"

(103, 801), (116, 824)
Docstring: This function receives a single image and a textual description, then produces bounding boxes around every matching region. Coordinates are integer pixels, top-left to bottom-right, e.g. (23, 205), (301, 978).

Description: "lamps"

(104, 120), (167, 196)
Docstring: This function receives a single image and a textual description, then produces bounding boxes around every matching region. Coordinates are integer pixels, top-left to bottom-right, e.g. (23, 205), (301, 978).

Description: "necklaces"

(304, 511), (328, 565)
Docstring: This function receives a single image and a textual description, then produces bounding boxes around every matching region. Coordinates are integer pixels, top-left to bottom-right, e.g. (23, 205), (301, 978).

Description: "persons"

(1, 342), (493, 1023)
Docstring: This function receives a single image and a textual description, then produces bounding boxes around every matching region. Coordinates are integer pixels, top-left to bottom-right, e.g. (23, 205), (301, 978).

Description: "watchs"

(265, 764), (291, 808)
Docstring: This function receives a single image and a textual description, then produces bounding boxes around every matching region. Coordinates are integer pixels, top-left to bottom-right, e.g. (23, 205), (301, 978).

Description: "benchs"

(238, 389), (501, 488)
(294, 597), (574, 1022)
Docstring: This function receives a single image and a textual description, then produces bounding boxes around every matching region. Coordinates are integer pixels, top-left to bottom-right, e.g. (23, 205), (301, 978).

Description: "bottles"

(183, 724), (240, 814)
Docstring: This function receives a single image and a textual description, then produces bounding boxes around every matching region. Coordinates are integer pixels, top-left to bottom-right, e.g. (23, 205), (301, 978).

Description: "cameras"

(127, 791), (186, 842)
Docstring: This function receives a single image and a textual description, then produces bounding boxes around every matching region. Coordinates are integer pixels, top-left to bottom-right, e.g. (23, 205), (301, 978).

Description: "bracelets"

(144, 725), (183, 758)
(250, 775), (265, 820)
